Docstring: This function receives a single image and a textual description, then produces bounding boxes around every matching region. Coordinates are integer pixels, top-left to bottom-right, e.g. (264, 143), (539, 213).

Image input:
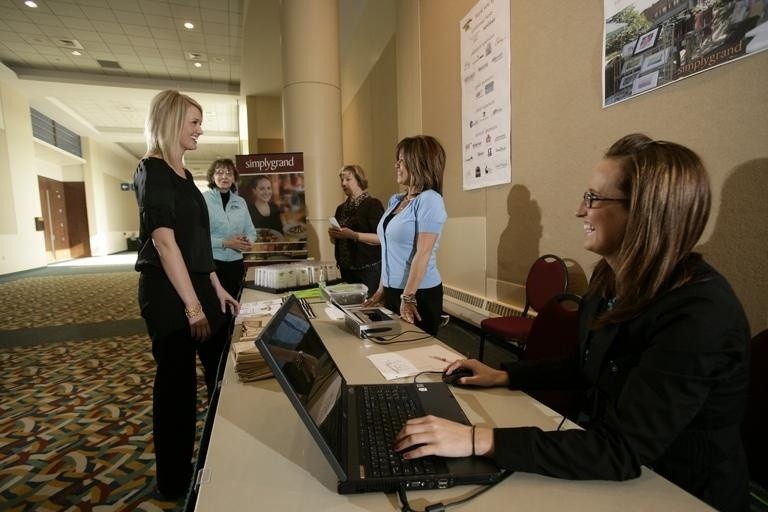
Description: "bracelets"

(472, 424), (475, 455)
(400, 294), (417, 306)
(222, 240), (225, 249)
(185, 303), (202, 317)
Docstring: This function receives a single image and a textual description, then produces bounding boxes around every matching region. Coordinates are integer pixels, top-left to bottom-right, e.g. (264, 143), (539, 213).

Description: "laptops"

(255, 293), (502, 494)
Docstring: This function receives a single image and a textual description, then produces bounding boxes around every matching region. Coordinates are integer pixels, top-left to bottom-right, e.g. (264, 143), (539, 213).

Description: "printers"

(331, 306), (401, 339)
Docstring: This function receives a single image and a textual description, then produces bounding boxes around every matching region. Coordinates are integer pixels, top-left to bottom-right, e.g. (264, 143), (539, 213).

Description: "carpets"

(0, 263), (225, 512)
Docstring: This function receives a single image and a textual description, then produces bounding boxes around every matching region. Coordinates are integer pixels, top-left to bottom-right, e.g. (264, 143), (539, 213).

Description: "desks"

(176, 262), (724, 511)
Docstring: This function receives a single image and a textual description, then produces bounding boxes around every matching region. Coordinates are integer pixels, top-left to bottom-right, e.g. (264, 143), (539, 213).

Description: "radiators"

(440, 282), (540, 354)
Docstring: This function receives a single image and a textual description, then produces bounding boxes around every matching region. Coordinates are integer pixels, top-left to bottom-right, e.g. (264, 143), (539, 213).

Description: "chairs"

(737, 326), (768, 511)
(518, 291), (607, 427)
(477, 252), (568, 367)
(126, 237), (139, 251)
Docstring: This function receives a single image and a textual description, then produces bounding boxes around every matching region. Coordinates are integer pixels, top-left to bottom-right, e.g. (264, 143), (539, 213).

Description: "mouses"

(442, 368), (477, 389)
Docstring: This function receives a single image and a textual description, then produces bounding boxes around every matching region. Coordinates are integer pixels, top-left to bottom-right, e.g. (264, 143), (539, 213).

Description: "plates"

(255, 228), (283, 241)
(283, 222), (307, 235)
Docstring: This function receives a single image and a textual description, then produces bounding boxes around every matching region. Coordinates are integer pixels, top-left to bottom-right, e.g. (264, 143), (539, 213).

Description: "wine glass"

(689, 31), (707, 58)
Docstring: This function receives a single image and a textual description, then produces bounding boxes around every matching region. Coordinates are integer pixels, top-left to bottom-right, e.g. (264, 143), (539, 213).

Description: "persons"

(360, 136), (448, 338)
(202, 158), (257, 299)
(328, 164), (385, 299)
(248, 175), (283, 234)
(395, 133), (751, 512)
(133, 89), (240, 502)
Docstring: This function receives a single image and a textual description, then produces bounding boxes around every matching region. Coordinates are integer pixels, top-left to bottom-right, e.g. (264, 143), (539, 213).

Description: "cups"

(679, 50), (687, 65)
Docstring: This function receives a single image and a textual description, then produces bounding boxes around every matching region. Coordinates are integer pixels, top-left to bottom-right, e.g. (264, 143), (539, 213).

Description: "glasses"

(217, 170), (233, 175)
(583, 192), (629, 208)
